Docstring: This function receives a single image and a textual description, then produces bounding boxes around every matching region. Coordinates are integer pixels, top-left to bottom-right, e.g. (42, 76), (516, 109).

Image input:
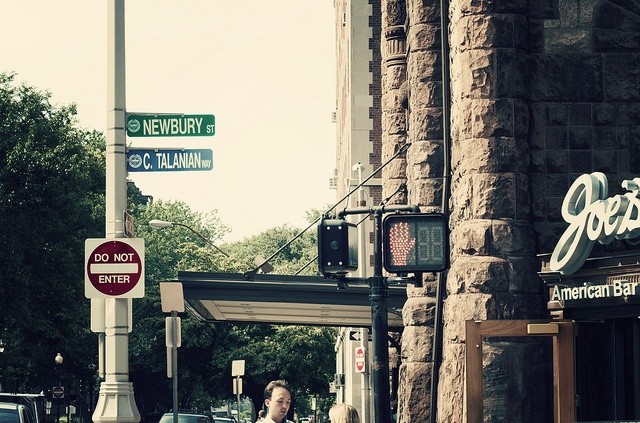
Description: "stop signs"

(84, 238), (145, 298)
(53, 387), (64, 398)
(355, 347), (365, 373)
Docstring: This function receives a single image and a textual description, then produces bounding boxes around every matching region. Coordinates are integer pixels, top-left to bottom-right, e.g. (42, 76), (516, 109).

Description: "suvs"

(211, 411), (228, 417)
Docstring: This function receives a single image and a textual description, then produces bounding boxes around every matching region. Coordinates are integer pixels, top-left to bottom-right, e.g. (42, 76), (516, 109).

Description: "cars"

(213, 418), (235, 423)
(0, 402), (33, 423)
(159, 413), (212, 423)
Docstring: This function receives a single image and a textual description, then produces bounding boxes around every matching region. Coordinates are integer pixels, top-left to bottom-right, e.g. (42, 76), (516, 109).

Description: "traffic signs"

(127, 113), (215, 137)
(126, 148), (213, 172)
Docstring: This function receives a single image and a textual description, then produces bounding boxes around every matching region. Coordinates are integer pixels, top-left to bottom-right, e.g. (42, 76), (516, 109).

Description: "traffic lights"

(384, 213), (451, 273)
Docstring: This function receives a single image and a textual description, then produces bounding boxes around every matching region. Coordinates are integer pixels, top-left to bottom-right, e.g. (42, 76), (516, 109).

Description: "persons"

(254, 409), (266, 423)
(253, 379), (294, 423)
(327, 403), (360, 422)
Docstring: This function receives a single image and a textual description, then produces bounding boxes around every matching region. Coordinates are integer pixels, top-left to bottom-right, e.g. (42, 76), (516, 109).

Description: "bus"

(0, 390), (44, 423)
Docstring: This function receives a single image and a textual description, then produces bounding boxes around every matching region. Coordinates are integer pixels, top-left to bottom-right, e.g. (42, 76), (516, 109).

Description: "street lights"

(88, 363), (96, 423)
(351, 162), (370, 423)
(55, 353), (64, 423)
